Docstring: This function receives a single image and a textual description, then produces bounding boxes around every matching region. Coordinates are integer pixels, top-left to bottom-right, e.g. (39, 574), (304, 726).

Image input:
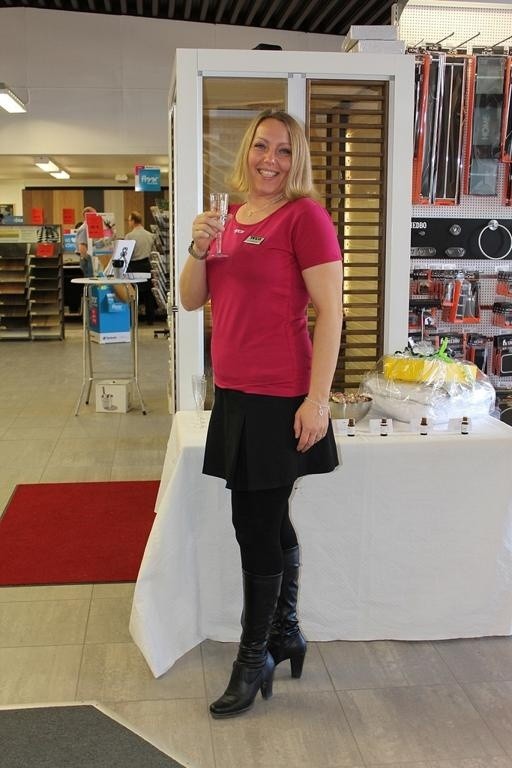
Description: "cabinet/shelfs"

(419, 417), (428, 435)
(347, 418), (355, 437)
(461, 416), (468, 435)
(380, 418), (388, 436)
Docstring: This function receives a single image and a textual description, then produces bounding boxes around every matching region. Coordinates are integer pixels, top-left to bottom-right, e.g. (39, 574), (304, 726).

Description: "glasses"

(0, 89), (27, 114)
(49, 170), (70, 180)
(33, 157), (60, 173)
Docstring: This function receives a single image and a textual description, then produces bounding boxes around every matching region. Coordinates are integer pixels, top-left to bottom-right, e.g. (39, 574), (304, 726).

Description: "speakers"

(210, 568), (283, 719)
(267, 544), (306, 678)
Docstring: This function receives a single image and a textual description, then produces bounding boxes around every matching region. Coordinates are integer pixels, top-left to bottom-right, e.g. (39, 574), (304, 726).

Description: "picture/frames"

(129, 410), (512, 680)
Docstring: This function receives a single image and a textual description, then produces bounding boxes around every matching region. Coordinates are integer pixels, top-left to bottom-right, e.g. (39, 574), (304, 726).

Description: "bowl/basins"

(246, 194), (285, 217)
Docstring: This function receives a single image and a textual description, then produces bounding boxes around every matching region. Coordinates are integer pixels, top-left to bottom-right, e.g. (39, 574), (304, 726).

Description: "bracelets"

(328, 395), (373, 423)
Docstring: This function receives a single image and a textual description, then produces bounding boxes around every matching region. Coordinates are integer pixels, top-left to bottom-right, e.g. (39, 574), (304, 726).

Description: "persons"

(178, 109), (344, 716)
(76, 207), (97, 278)
(124, 210), (155, 326)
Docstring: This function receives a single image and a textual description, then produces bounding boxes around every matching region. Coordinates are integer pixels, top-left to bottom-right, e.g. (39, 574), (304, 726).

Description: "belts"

(207, 192), (230, 259)
(190, 374), (207, 430)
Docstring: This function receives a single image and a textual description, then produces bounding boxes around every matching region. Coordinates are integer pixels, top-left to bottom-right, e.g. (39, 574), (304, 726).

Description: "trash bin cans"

(188, 240), (210, 260)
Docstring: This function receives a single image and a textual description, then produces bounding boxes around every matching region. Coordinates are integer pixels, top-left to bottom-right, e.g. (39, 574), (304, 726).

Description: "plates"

(0, 224), (65, 341)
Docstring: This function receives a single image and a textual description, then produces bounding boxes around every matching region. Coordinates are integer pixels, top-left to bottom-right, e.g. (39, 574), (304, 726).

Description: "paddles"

(0, 480), (161, 587)
(0, 705), (187, 768)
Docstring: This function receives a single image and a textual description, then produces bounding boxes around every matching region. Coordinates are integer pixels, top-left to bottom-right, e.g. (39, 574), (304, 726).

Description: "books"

(95, 379), (133, 413)
(37, 243), (58, 256)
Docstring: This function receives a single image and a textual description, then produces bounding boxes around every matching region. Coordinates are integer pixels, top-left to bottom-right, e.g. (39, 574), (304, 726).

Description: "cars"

(111, 259), (124, 279)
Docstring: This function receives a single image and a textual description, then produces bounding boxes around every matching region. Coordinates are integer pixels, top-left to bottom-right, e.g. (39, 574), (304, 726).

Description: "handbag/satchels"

(305, 397), (331, 419)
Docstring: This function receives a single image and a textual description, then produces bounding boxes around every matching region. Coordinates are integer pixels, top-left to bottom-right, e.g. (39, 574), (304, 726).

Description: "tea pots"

(0, 154), (169, 254)
